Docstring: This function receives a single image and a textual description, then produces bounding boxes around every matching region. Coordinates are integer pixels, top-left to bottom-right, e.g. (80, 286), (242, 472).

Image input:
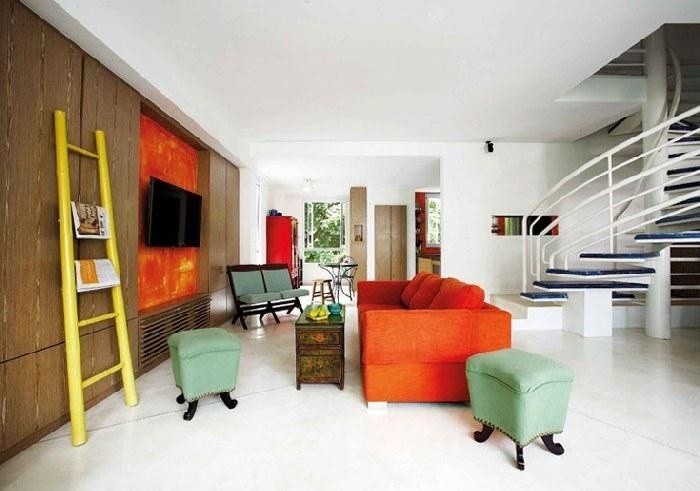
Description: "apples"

(307, 305), (327, 318)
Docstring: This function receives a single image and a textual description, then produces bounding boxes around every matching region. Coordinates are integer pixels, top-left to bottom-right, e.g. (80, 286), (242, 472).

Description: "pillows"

(401, 269), (485, 311)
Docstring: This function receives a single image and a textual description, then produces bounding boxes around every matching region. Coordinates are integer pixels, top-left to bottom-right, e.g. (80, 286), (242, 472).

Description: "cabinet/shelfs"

(267, 216), (303, 289)
(296, 302), (345, 391)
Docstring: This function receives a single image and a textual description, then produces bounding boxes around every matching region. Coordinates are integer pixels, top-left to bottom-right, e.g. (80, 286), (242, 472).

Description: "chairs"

(226, 263), (310, 331)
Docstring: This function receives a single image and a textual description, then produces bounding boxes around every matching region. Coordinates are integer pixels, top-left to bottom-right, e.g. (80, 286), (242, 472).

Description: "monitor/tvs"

(143, 175), (204, 250)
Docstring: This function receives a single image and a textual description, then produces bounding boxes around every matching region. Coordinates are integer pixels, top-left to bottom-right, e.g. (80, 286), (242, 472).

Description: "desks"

(321, 262), (358, 301)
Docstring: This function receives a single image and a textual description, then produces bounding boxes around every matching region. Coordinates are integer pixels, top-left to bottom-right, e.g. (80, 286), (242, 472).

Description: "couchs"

(357, 279), (513, 410)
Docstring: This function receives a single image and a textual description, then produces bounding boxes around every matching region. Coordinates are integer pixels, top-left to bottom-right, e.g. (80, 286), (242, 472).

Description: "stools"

(167, 327), (238, 420)
(465, 346), (571, 470)
(311, 278), (335, 305)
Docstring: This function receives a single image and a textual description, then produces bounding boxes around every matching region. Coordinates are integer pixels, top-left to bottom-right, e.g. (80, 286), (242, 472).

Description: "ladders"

(54, 110), (138, 447)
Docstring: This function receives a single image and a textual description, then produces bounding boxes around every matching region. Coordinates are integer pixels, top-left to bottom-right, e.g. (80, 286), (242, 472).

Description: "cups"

(328, 304), (342, 314)
